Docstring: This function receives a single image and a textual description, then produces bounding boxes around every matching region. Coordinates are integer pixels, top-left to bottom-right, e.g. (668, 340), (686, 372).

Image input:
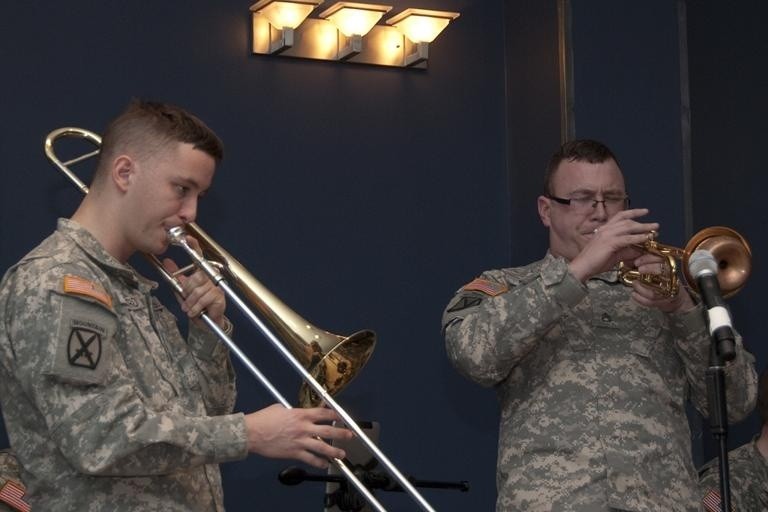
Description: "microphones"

(687, 248), (737, 361)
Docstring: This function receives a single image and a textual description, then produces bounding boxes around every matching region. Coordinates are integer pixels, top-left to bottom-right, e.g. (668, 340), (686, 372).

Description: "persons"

(0, 95), (353, 512)
(440, 138), (758, 512)
(695, 420), (768, 512)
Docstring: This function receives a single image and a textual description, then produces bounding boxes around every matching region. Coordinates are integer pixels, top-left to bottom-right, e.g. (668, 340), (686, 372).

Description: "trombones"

(44, 127), (437, 512)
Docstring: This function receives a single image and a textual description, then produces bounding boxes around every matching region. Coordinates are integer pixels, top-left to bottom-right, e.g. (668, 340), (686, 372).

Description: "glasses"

(547, 195), (631, 210)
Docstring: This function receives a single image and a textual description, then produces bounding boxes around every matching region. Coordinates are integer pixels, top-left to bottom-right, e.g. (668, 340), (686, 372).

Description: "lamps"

(249, 0), (461, 72)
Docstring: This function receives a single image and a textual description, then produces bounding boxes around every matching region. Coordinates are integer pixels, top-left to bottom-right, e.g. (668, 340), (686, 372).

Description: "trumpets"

(588, 221), (752, 303)
